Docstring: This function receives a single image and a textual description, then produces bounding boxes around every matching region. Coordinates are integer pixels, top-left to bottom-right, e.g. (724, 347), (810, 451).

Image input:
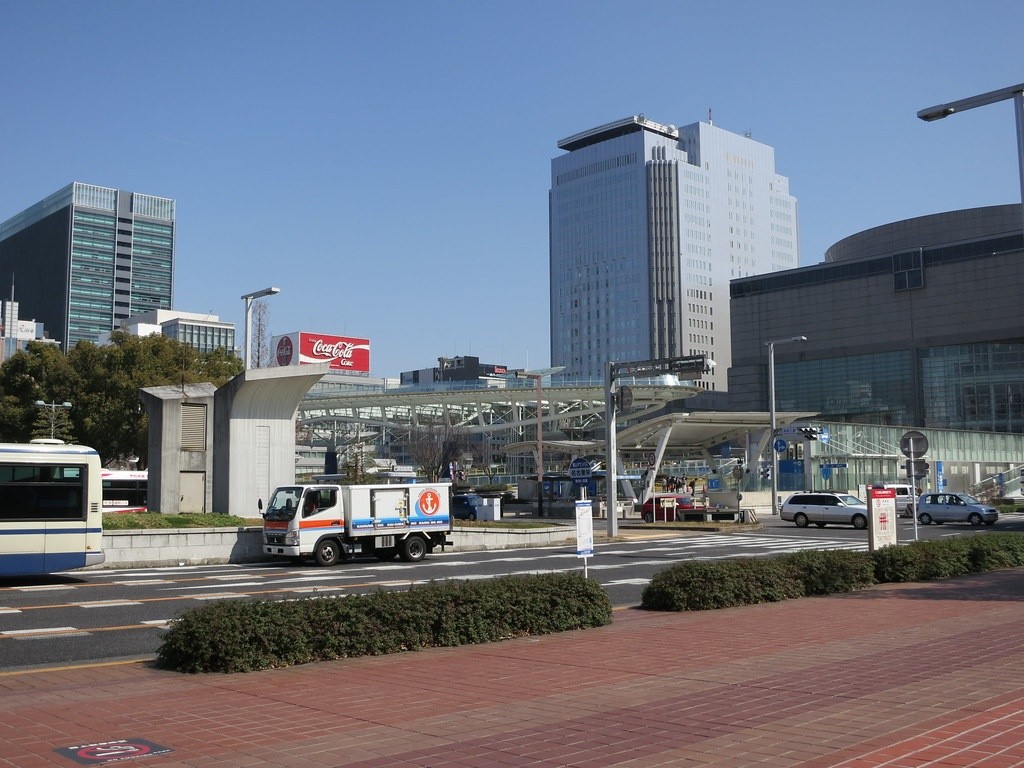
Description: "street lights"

(515, 371), (544, 518)
(240, 286), (280, 369)
(915, 83), (1024, 217)
(35, 399), (72, 439)
(763, 335), (808, 515)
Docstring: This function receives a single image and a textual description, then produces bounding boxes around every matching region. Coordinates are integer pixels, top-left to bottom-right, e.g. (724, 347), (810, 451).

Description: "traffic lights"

(764, 467), (770, 478)
(798, 427), (820, 440)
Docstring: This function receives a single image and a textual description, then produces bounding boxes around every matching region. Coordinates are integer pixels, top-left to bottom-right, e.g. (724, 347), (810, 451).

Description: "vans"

(872, 484), (922, 512)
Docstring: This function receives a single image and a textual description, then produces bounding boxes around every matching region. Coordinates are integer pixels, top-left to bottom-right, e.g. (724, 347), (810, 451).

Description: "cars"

(452, 494), (483, 521)
(641, 495), (706, 523)
(916, 492), (997, 527)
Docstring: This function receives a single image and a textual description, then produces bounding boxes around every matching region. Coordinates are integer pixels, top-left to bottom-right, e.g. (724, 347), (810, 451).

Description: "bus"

(64, 467), (149, 516)
(0, 438), (105, 577)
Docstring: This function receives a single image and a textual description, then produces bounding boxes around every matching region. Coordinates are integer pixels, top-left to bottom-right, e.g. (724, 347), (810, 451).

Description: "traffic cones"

(702, 485), (706, 493)
(686, 484), (690, 492)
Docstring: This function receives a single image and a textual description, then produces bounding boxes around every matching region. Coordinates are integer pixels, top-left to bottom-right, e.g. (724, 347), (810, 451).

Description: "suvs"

(780, 489), (868, 530)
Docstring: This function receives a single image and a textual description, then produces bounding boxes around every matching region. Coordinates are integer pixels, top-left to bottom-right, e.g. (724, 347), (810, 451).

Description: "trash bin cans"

(477, 497), (500, 521)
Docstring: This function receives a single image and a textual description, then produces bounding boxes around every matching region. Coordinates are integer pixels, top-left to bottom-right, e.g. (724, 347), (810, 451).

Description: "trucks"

(258, 483), (453, 566)
